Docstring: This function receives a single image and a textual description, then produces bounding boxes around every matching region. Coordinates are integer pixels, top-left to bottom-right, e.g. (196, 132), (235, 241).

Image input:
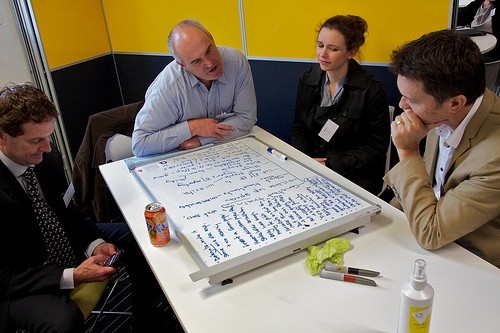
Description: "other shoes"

(129, 310), (166, 332)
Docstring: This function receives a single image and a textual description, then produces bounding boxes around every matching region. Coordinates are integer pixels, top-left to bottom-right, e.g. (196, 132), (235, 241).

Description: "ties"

(21, 167), (77, 270)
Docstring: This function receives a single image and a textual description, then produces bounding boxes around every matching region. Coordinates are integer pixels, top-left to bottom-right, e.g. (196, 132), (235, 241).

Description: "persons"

(131, 20), (257, 157)
(383, 28), (500, 270)
(291, 14), (390, 196)
(0, 81), (174, 333)
(457, 0), (500, 63)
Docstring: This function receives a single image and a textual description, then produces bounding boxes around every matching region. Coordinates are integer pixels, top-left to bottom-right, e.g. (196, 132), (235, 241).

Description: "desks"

(468, 33), (497, 55)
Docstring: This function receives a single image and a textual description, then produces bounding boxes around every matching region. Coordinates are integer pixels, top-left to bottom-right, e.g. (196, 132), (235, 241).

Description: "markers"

(319, 270), (377, 286)
(267, 147), (288, 160)
(325, 264), (380, 277)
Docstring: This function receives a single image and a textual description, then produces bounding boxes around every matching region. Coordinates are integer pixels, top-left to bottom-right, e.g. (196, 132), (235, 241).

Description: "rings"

(397, 121), (404, 125)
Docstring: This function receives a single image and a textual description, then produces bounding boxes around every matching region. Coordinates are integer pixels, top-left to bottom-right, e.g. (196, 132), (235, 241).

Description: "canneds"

(144, 202), (171, 247)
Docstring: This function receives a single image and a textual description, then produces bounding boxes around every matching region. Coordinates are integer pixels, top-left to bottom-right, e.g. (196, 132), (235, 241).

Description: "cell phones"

(104, 247), (125, 267)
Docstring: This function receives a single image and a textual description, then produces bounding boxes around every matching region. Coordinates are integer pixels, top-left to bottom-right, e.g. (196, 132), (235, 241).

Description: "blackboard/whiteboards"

(124, 134), (382, 286)
(0, 0), (58, 92)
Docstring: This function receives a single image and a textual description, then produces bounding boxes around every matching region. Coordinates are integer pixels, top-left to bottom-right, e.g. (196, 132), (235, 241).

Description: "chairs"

(71, 279), (133, 333)
(98, 124), (500, 333)
(89, 100), (145, 222)
